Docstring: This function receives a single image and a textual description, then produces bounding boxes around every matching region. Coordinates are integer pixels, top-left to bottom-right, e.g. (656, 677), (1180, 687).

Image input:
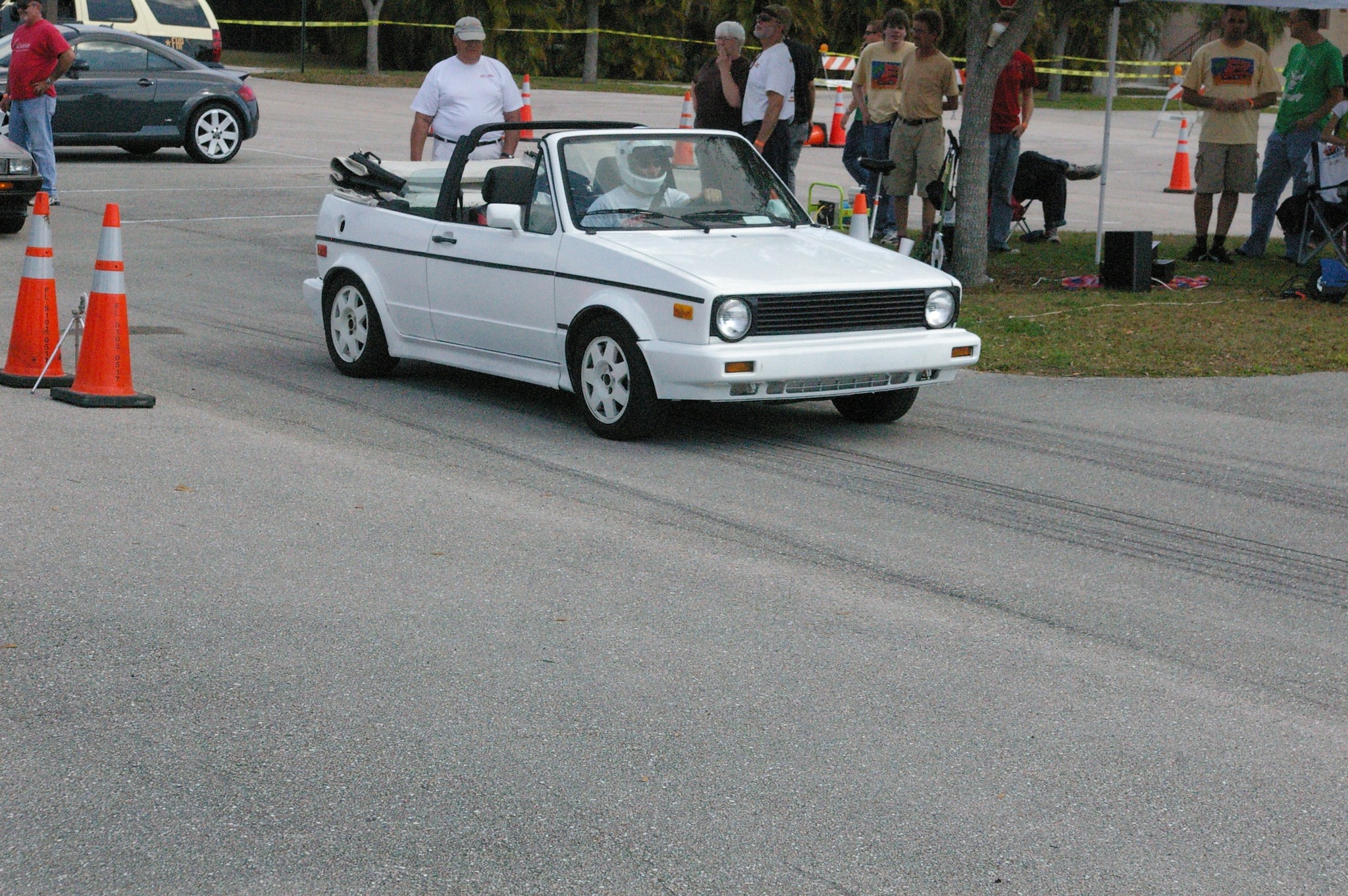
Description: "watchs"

(45, 78), (53, 85)
(501, 153), (515, 159)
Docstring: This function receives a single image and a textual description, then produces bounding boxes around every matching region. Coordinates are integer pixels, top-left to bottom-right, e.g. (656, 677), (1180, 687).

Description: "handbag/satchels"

(1276, 193), (1312, 245)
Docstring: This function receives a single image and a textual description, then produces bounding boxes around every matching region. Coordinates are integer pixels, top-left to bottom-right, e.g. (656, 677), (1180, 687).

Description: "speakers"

(1105, 231), (1153, 292)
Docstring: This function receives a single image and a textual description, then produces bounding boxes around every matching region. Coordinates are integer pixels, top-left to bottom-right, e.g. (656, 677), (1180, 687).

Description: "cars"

(0, 133), (44, 235)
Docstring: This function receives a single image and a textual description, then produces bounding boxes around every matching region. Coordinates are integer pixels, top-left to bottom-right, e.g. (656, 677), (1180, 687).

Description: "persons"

(0, 0), (75, 207)
(579, 126), (723, 227)
(840, 9), (917, 246)
(963, 11), (1103, 255)
(411, 15), (525, 162)
(1180, 5), (1282, 264)
(690, 21), (749, 202)
(887, 8), (960, 255)
(741, 4), (816, 198)
(1235, 8), (1348, 257)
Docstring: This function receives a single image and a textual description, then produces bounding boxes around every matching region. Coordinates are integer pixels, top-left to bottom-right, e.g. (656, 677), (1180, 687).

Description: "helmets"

(1311, 260), (1348, 300)
(616, 126), (674, 194)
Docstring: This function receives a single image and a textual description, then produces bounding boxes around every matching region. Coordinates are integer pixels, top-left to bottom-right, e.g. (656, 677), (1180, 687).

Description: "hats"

(454, 16), (485, 40)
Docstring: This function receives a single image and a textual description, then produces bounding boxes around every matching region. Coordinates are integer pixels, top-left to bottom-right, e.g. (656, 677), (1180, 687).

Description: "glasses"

(1227, 17), (1246, 25)
(714, 35), (738, 42)
(639, 159), (661, 168)
(757, 14), (778, 23)
(16, 3), (36, 10)
(910, 25), (930, 35)
(865, 30), (877, 35)
(1288, 18), (1304, 24)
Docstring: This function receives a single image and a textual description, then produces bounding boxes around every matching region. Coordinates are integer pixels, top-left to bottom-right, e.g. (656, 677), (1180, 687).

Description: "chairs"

(1294, 178), (1348, 266)
(946, 130), (1034, 243)
(470, 165), (553, 229)
(592, 154), (618, 198)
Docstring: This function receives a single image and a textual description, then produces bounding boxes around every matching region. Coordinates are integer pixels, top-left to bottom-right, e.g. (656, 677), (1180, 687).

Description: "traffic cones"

(1163, 119), (1196, 194)
(50, 204), (156, 409)
(670, 89), (701, 169)
(520, 74), (539, 142)
(829, 87), (846, 147)
(801, 122), (829, 148)
(848, 194), (871, 243)
(0, 190), (76, 388)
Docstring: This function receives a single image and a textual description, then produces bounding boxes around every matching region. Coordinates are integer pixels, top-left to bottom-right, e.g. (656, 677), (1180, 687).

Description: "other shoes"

(1229, 248), (1252, 257)
(1045, 227), (1061, 245)
(1182, 244), (1207, 262)
(996, 247), (1020, 256)
(1282, 255), (1296, 262)
(1067, 163), (1102, 180)
(1209, 246), (1232, 262)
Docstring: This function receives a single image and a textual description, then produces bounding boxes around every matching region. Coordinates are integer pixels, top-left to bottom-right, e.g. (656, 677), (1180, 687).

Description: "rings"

(39, 88), (41, 90)
(715, 197), (719, 199)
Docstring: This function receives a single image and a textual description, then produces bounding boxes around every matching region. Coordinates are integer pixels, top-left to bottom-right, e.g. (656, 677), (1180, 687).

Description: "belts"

(433, 133), (502, 147)
(898, 116), (939, 126)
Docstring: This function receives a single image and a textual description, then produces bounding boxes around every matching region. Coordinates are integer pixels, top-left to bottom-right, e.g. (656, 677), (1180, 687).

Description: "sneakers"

(880, 228), (899, 247)
(873, 230), (883, 237)
(29, 195), (60, 206)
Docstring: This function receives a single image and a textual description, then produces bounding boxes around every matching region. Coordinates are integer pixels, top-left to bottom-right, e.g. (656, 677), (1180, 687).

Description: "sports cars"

(0, 24), (261, 166)
(306, 119), (982, 442)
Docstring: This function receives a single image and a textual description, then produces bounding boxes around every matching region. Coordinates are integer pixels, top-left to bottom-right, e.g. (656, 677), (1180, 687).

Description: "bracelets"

(1246, 99), (1254, 109)
(754, 139), (765, 147)
(3, 93), (12, 100)
(845, 108), (851, 115)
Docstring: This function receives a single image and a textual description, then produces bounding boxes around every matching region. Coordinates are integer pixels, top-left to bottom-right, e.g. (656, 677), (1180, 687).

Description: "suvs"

(0, 0), (224, 70)
